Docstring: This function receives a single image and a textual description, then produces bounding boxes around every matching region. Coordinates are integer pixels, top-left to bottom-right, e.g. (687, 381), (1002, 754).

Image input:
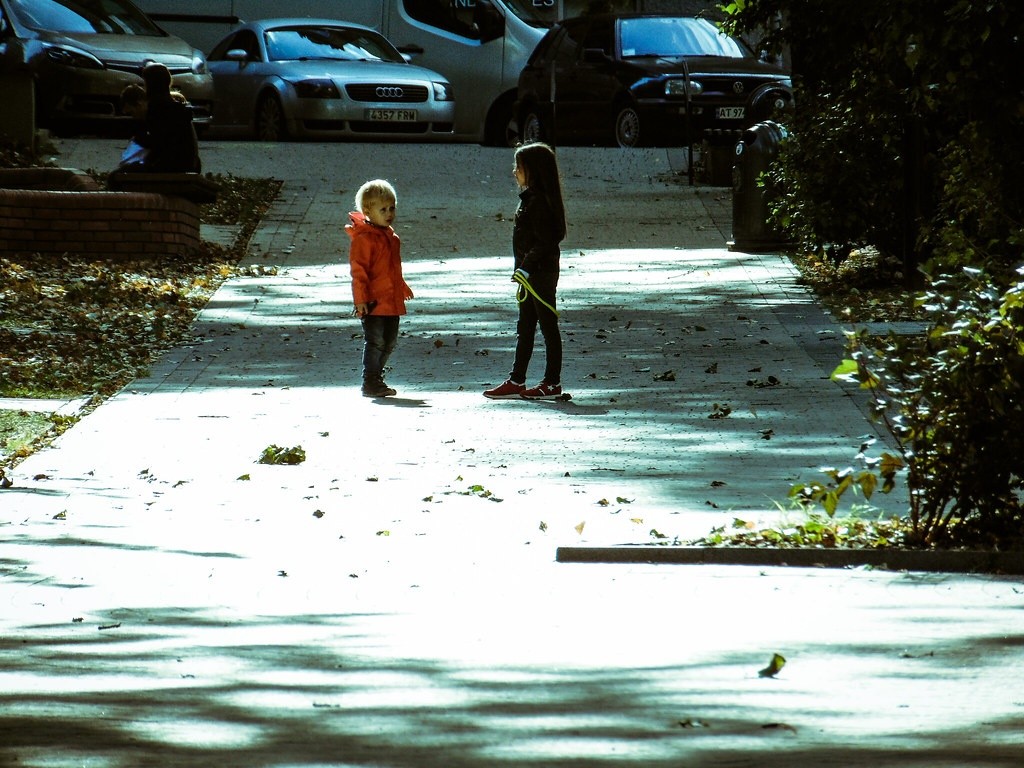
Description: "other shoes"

(361, 381), (397, 397)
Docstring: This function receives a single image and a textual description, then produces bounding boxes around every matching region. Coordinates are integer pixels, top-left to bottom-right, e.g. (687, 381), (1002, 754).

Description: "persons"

(106, 63), (202, 191)
(482, 143), (569, 401)
(344, 179), (414, 397)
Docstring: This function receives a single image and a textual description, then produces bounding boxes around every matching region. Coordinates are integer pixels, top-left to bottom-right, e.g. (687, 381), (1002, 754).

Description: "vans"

(127, 0), (567, 148)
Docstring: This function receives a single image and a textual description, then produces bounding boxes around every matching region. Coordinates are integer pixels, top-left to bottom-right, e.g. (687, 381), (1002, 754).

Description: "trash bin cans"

(733, 120), (787, 244)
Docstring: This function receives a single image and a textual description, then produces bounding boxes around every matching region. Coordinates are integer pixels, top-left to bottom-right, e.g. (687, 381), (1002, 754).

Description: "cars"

(208, 14), (461, 141)
(515, 12), (799, 150)
(2, 1), (215, 129)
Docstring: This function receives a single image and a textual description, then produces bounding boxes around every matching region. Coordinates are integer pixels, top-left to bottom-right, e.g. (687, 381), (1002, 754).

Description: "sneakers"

(482, 379), (526, 399)
(520, 382), (562, 400)
